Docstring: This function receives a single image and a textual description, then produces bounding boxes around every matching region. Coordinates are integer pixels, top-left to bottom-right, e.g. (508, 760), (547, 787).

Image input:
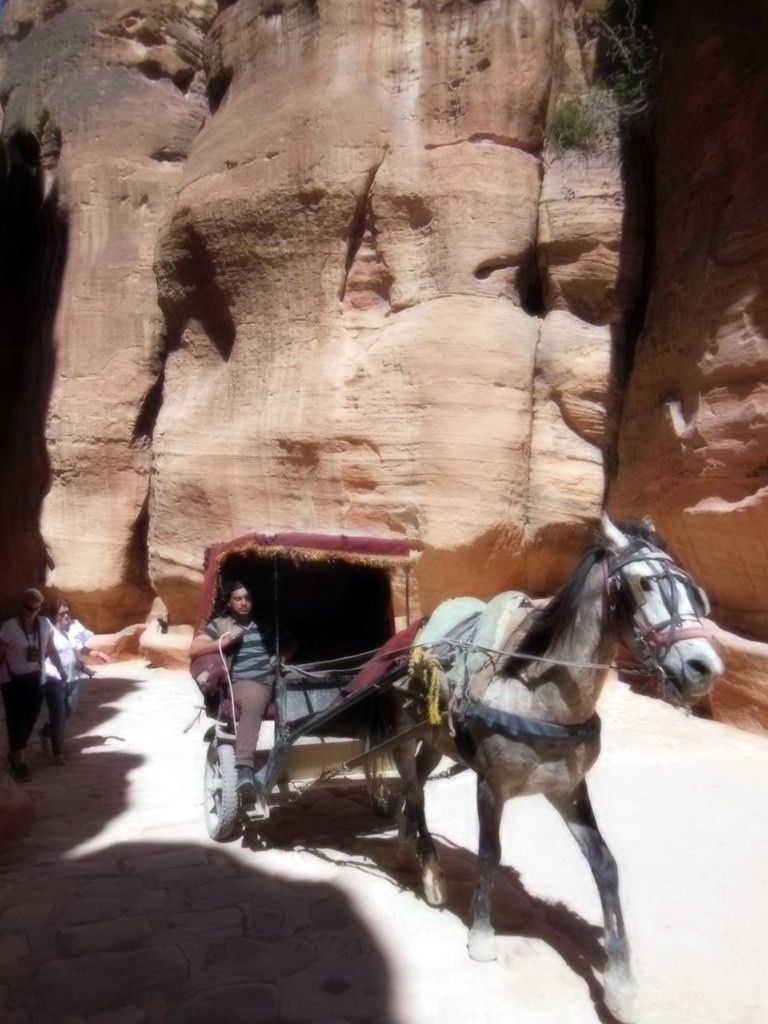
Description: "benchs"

(220, 626), (389, 750)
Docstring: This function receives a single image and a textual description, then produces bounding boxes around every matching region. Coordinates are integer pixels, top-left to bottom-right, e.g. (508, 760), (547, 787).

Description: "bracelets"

(60, 669), (65, 674)
(95, 651), (100, 658)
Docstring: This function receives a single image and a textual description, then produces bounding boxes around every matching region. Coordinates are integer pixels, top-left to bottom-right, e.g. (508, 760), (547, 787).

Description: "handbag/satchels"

(11, 671), (41, 692)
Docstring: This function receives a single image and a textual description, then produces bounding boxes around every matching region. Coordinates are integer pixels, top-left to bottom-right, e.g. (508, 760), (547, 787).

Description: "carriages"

(185, 511), (726, 1024)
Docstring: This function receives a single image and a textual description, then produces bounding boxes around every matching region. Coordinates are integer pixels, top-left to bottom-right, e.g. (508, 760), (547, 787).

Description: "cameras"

(27, 646), (38, 663)
(75, 662), (97, 679)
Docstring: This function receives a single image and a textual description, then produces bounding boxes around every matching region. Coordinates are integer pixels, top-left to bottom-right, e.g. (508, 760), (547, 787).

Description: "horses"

(363, 507), (725, 1024)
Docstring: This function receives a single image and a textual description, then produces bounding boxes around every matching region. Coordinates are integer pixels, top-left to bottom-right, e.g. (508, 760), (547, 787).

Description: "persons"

(36, 597), (111, 771)
(0, 588), (55, 782)
(190, 581), (299, 793)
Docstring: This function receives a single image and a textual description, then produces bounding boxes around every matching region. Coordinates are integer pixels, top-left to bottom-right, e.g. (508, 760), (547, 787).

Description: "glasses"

(25, 604), (42, 612)
(58, 611), (71, 617)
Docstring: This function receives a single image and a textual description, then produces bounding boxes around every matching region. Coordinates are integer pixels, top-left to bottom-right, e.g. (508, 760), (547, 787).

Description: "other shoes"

(37, 727), (52, 753)
(7, 751), (32, 782)
(235, 767), (256, 794)
(48, 748), (67, 773)
